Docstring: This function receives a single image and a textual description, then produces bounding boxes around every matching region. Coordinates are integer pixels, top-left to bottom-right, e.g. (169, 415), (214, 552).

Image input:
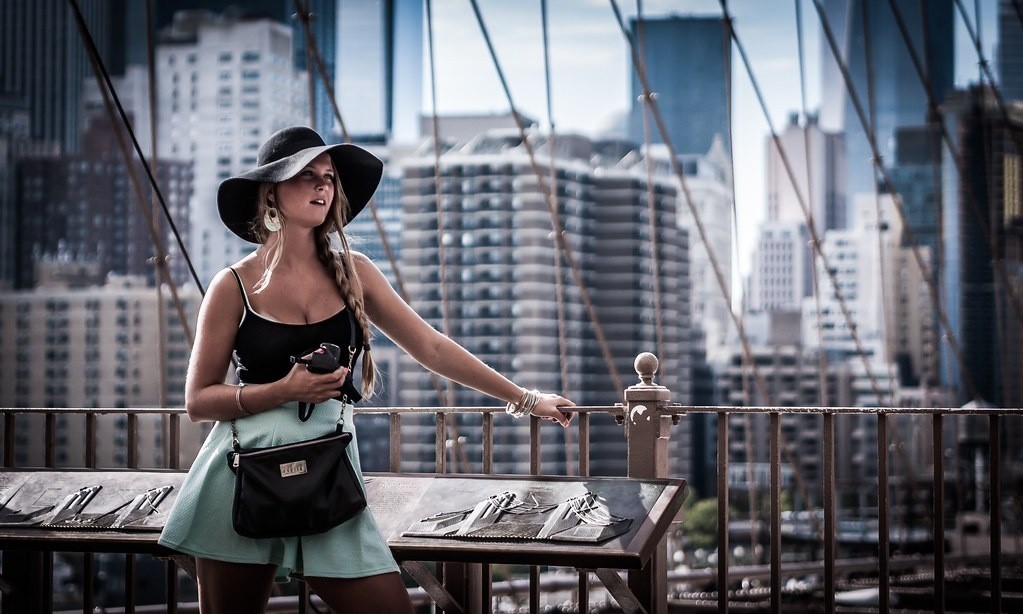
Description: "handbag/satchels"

(227, 423), (369, 540)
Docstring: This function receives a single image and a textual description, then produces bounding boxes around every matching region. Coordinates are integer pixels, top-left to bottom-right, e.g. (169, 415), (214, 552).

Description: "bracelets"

(236, 385), (251, 416)
(506, 388), (540, 418)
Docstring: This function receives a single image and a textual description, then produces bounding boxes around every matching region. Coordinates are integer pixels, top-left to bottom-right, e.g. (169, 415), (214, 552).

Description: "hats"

(214, 125), (386, 245)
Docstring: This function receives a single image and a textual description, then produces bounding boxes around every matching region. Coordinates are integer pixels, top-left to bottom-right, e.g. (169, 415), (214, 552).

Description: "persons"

(159, 127), (576, 614)
